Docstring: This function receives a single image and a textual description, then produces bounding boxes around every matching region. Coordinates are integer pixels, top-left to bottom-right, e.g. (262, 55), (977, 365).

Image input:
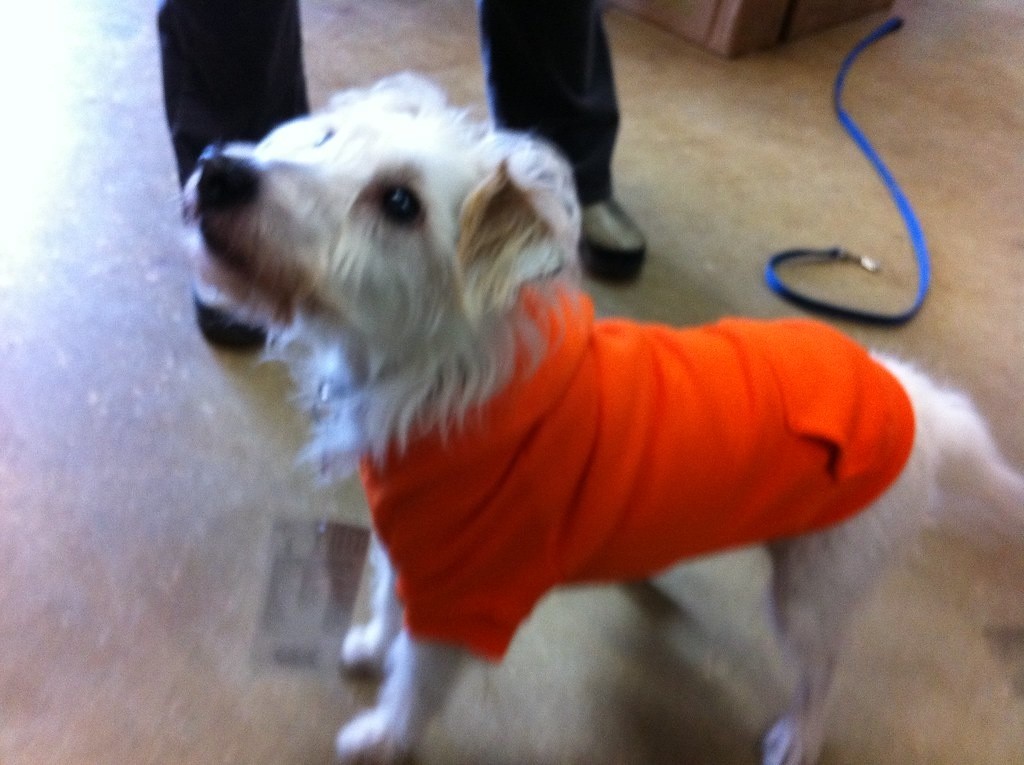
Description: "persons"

(160, 2), (650, 354)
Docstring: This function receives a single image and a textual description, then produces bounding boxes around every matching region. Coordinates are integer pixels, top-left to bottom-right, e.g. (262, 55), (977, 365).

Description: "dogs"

(179, 71), (1024, 764)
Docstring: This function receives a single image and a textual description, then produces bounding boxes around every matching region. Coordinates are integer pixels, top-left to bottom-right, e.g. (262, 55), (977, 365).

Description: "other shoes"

(196, 299), (265, 349)
(579, 194), (646, 272)
